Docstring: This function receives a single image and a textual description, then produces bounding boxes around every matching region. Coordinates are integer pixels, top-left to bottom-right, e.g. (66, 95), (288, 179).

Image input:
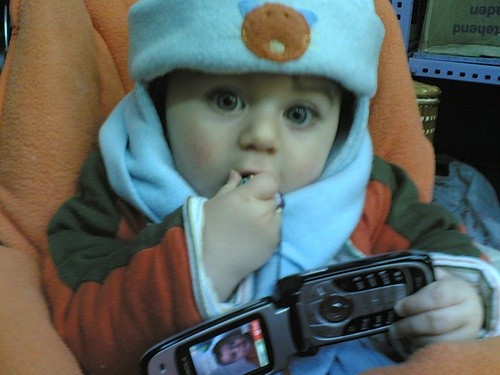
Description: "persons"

(214, 331), (258, 367)
(40, 0), (499, 375)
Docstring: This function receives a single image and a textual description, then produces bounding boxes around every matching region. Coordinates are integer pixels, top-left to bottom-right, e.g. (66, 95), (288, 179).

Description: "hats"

(128, 1), (385, 99)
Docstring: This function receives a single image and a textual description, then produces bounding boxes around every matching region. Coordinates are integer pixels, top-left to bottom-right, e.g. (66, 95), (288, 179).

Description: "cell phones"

(140, 250), (436, 375)
